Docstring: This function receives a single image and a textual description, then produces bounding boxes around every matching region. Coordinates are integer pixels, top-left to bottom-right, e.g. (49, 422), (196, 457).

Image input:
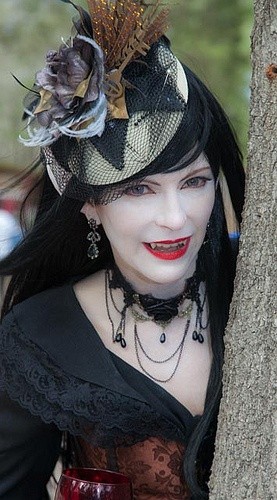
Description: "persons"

(1, 0), (245, 500)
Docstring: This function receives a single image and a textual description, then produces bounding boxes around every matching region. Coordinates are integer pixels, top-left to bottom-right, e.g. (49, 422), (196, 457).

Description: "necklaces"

(103, 258), (212, 383)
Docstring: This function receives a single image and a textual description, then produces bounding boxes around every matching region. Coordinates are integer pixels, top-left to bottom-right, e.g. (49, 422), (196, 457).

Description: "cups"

(52, 468), (133, 500)
